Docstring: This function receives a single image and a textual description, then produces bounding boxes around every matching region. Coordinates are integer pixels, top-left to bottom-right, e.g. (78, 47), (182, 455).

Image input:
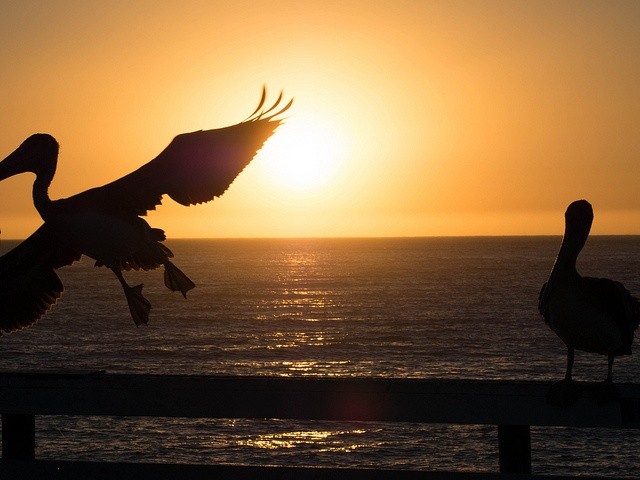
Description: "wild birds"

(535, 195), (640, 386)
(1, 83), (298, 336)
